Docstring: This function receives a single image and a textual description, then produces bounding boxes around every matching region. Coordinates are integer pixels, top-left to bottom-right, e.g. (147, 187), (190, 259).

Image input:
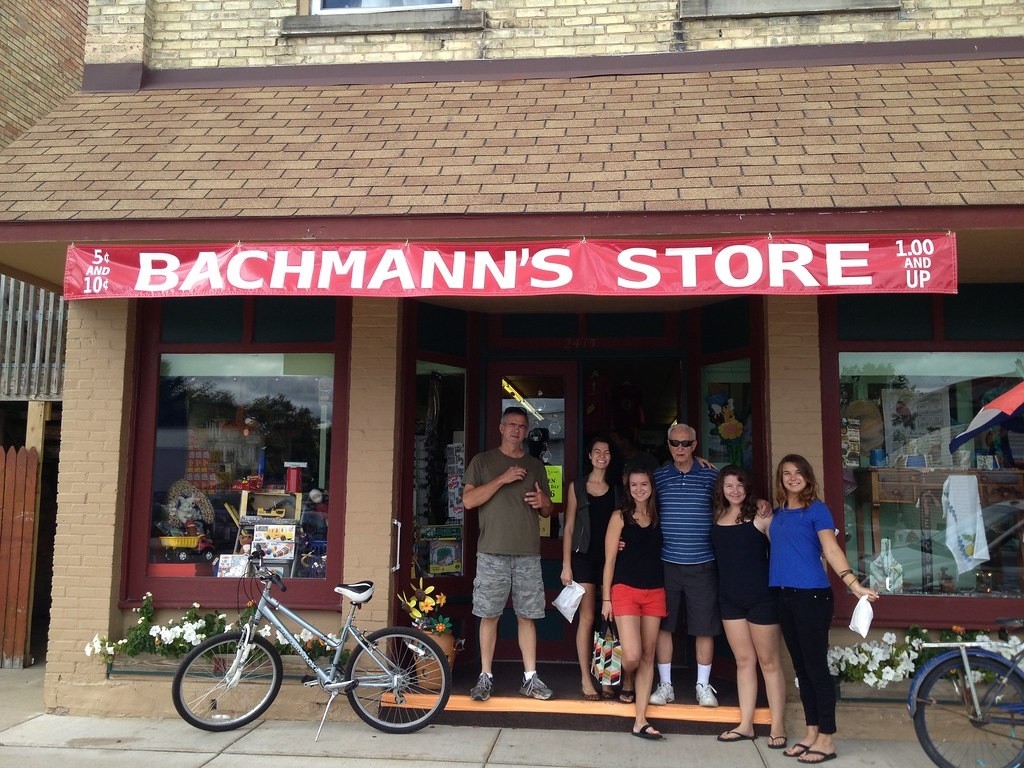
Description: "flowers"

(793, 623), (1024, 704)
(395, 582), (453, 637)
(85, 589), (351, 670)
(706, 393), (750, 445)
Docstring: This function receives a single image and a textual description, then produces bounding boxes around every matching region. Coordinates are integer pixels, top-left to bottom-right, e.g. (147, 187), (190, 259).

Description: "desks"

(852, 465), (1024, 598)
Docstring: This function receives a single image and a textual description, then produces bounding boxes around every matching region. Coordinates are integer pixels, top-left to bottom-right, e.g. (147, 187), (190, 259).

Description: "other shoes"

(580, 689), (601, 700)
(602, 691), (617, 700)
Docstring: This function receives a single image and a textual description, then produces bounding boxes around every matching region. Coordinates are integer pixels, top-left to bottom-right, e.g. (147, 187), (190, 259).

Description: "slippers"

(797, 749), (837, 763)
(768, 736), (787, 748)
(716, 730), (757, 742)
(618, 690), (635, 704)
(632, 724), (663, 739)
(783, 743), (810, 756)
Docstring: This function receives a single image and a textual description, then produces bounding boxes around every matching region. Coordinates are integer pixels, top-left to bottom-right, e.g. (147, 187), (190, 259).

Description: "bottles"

(287, 466), (302, 493)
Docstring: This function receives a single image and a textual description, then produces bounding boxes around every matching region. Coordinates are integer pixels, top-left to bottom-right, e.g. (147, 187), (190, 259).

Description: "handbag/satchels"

(590, 617), (622, 685)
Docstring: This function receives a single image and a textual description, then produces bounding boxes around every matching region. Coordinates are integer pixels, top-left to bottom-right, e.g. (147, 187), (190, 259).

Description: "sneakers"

(648, 682), (675, 705)
(519, 673), (554, 700)
(470, 673), (494, 700)
(695, 683), (719, 706)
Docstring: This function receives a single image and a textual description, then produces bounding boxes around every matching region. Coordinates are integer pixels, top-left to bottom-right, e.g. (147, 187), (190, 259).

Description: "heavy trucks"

(158, 533), (216, 562)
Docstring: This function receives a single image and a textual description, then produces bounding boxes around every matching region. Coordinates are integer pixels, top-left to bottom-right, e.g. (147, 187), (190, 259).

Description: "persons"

(601, 424), (879, 764)
(560, 438), (628, 702)
(462, 407), (556, 701)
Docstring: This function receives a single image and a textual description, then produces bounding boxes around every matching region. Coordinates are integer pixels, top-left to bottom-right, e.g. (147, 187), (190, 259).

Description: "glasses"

(668, 440), (694, 447)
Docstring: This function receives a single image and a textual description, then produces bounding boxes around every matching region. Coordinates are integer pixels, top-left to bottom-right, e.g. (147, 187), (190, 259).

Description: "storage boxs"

(302, 540), (327, 578)
(418, 524), (463, 573)
(217, 489), (303, 579)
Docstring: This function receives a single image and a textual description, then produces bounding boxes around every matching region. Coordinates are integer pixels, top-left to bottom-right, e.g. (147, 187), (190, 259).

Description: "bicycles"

(172, 544), (452, 733)
(904, 615), (1024, 768)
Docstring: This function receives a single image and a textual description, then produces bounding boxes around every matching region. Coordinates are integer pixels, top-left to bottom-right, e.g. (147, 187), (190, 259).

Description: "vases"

(729, 442), (744, 471)
(412, 629), (457, 690)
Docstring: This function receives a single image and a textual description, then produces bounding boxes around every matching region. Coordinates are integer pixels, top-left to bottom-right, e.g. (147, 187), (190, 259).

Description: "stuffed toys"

(237, 534), (253, 554)
(178, 496), (203, 523)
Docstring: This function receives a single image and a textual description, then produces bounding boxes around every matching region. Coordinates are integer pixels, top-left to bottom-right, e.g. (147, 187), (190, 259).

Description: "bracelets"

(840, 569), (853, 581)
(603, 600), (610, 602)
(848, 578), (857, 588)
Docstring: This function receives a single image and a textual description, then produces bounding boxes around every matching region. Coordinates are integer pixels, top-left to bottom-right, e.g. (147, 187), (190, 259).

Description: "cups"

(954, 450), (971, 470)
(869, 448), (890, 467)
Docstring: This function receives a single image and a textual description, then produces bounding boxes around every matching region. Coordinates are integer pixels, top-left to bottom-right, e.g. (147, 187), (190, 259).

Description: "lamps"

(502, 379), (561, 464)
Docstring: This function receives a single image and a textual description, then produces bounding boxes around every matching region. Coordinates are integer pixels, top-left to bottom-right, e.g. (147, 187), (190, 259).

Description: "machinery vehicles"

(257, 497), (295, 518)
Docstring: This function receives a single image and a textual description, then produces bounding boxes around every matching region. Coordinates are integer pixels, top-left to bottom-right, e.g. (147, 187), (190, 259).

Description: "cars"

(850, 498), (1024, 593)
